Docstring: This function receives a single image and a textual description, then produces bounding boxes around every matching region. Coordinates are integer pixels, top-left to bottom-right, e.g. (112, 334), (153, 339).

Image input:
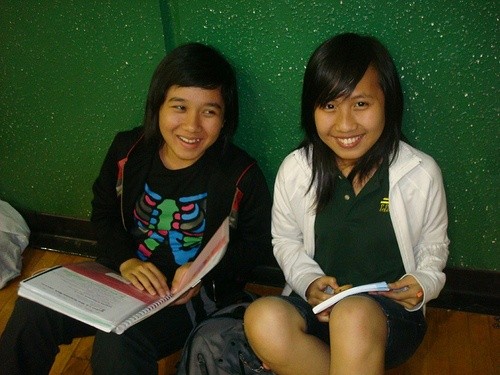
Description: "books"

(18, 217), (230, 335)
(312, 281), (389, 314)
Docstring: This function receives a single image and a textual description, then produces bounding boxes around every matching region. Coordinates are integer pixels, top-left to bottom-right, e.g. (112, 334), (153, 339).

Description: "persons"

(243, 32), (451, 375)
(0, 43), (271, 375)
